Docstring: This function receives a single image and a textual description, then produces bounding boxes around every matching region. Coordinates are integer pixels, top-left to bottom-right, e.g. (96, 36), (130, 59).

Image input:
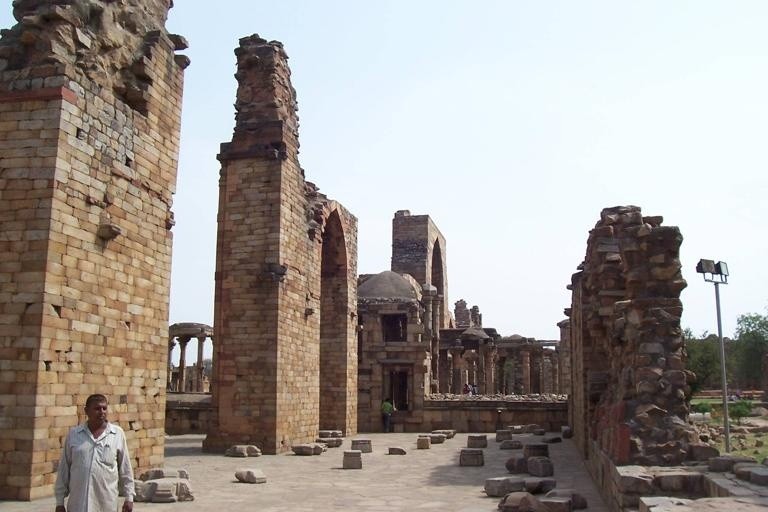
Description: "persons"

(462, 383), (469, 394)
(379, 396), (395, 433)
(467, 381), (474, 395)
(53, 393), (138, 512)
